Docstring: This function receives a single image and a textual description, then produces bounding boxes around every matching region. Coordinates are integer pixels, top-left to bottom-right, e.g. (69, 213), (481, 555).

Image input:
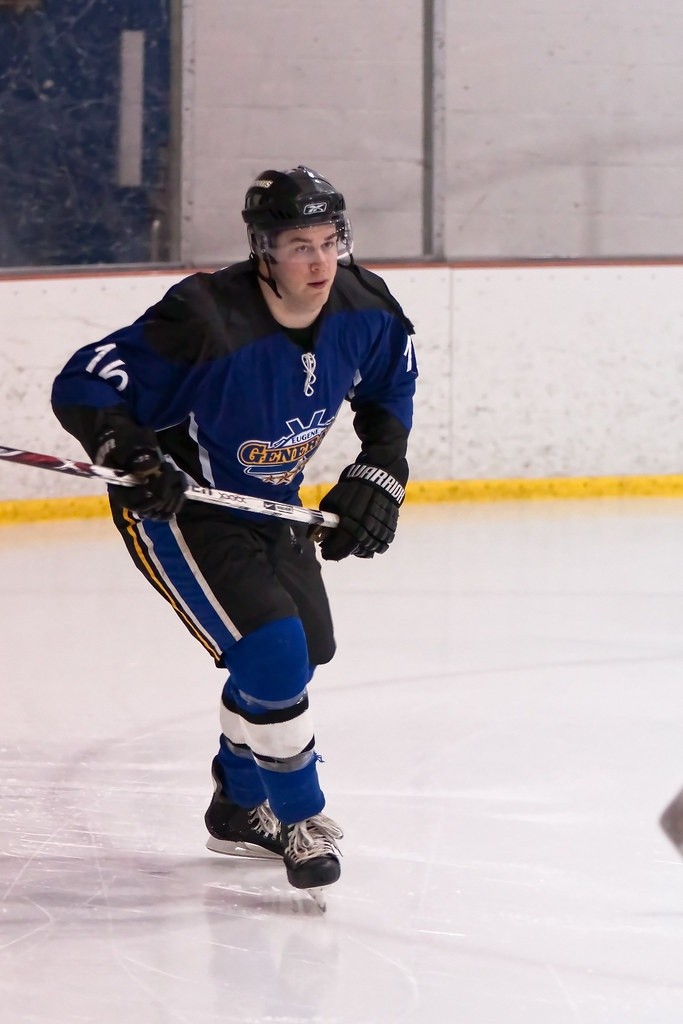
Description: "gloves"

(317, 449), (408, 563)
(91, 423), (188, 525)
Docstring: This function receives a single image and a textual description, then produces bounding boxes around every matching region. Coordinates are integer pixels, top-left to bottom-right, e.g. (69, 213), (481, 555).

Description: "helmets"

(241, 165), (345, 251)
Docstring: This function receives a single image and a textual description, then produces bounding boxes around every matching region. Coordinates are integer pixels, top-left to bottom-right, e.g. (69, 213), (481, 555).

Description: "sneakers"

(204, 791), (285, 860)
(279, 812), (345, 912)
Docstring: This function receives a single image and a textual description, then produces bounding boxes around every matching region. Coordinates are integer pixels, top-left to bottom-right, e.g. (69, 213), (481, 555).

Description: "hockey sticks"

(0, 430), (345, 533)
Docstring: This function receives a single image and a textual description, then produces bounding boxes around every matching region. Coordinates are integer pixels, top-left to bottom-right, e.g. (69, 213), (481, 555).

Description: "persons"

(50, 166), (418, 890)
